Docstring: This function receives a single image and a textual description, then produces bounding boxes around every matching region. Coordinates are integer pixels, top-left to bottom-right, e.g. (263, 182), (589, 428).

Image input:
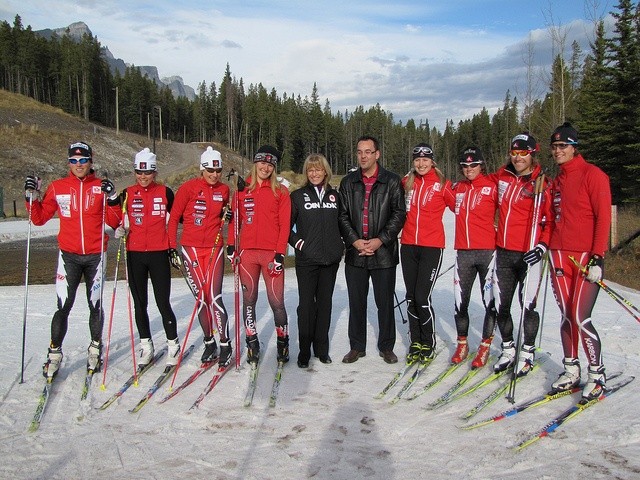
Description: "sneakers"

(200, 336), (218, 363)
(550, 356), (582, 392)
(164, 336), (181, 367)
(41, 347), (63, 377)
(419, 343), (435, 363)
(581, 363), (612, 400)
(85, 340), (103, 372)
(276, 336), (291, 363)
(471, 338), (493, 368)
(244, 333), (260, 364)
(137, 338), (155, 367)
(218, 338), (232, 367)
(406, 341), (420, 363)
(451, 334), (470, 364)
(491, 341), (517, 373)
(513, 344), (536, 377)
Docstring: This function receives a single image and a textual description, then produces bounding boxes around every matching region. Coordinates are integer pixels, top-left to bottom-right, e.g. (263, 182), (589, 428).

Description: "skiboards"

(100, 342), (195, 414)
(156, 356), (236, 413)
(373, 348), (442, 405)
(460, 372), (635, 451)
(403, 352), (497, 407)
(27, 372), (96, 432)
(243, 361), (284, 407)
(427, 348), (552, 420)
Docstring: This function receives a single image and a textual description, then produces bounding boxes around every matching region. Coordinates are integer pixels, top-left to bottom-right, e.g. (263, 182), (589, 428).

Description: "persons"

(112, 148), (183, 368)
(338, 135), (406, 363)
(227, 144), (292, 362)
(25, 142), (122, 381)
(446, 146), (498, 367)
(485, 132), (554, 376)
(402, 142), (455, 363)
(167, 146), (233, 367)
(287, 153), (344, 368)
(548, 122), (612, 400)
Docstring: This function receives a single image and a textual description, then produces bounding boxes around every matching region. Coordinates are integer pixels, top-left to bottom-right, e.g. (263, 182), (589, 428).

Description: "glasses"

(68, 157), (91, 164)
(510, 151), (533, 157)
(412, 146), (433, 155)
(549, 144), (569, 150)
(355, 149), (376, 157)
(203, 166), (222, 173)
(134, 169), (156, 175)
(460, 162), (482, 168)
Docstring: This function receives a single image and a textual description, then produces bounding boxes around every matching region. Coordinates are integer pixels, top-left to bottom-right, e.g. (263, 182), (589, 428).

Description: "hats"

(549, 121), (579, 148)
(459, 146), (484, 164)
(411, 142), (434, 161)
(199, 145), (223, 171)
(132, 147), (157, 171)
(510, 132), (537, 156)
(253, 144), (280, 168)
(67, 142), (93, 163)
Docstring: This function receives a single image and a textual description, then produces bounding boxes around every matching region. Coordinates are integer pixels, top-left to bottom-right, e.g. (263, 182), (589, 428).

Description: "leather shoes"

(297, 357), (309, 368)
(314, 352), (332, 364)
(341, 348), (367, 363)
(378, 349), (400, 364)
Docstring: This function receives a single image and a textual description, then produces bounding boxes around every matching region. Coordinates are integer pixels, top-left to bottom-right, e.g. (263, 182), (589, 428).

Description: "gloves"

(582, 255), (603, 285)
(24, 174), (41, 202)
(267, 253), (285, 279)
(221, 205), (233, 223)
(168, 248), (183, 270)
(523, 244), (546, 267)
(100, 178), (116, 197)
(225, 245), (242, 271)
